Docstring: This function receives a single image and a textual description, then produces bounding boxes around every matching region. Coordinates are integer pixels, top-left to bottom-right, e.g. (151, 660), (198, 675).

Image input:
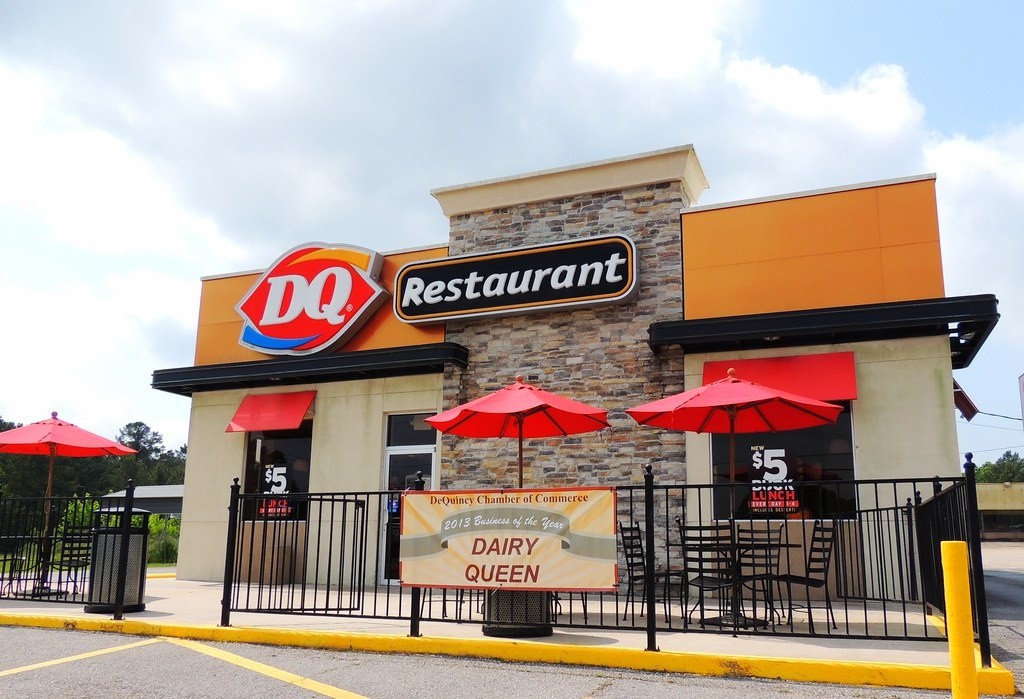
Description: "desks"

(685, 544), (802, 629)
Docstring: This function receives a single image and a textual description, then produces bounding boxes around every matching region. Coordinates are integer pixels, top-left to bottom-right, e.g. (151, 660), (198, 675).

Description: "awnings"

(702, 351), (858, 401)
(953, 377), (978, 422)
(225, 390), (317, 432)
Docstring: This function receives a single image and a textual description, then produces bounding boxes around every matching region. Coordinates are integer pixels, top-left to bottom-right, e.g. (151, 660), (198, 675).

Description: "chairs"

(43, 521), (94, 601)
(616, 517), (842, 629)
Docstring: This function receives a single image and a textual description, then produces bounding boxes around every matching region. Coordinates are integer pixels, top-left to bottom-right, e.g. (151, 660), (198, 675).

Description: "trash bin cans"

(480, 588), (558, 638)
(84, 506), (154, 613)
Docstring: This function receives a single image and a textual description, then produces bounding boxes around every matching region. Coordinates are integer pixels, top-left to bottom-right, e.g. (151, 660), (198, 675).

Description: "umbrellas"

(624, 369), (844, 520)
(424, 375), (612, 488)
(0, 412), (138, 535)
